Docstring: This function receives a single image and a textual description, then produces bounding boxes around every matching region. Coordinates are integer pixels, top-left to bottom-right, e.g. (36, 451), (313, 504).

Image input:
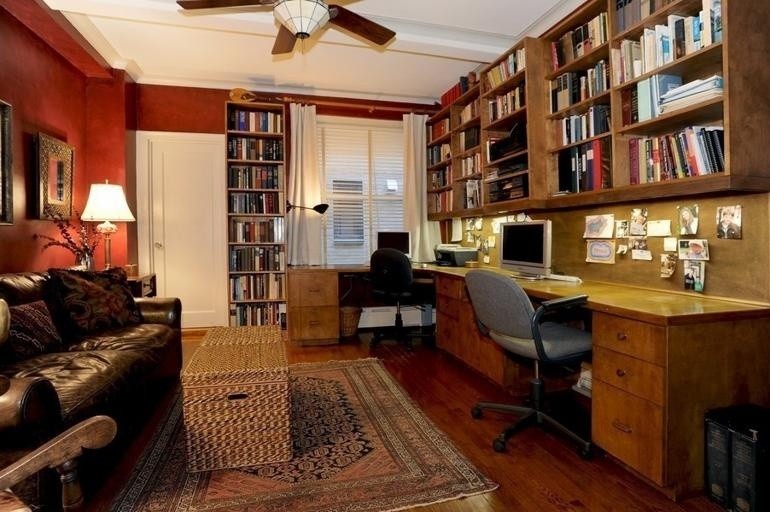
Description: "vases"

(80, 254), (94, 269)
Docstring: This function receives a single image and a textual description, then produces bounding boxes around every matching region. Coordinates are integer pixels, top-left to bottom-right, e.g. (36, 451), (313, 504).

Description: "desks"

(127, 273), (156, 298)
(287, 268), (770, 502)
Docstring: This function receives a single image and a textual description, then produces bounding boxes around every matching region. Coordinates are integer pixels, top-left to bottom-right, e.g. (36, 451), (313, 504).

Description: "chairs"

(1, 415), (117, 512)
(464, 272), (596, 458)
(368, 247), (435, 351)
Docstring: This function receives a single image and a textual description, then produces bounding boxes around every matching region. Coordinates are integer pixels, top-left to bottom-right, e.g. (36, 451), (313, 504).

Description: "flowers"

(32, 204), (100, 256)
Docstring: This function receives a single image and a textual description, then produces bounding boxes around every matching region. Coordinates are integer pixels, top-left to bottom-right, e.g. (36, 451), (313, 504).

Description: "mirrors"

(31, 129), (76, 221)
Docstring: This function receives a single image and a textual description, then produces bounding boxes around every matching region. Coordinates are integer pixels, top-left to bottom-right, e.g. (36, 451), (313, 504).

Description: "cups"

(421, 264), (427, 268)
(126, 265), (138, 279)
(465, 261), (479, 268)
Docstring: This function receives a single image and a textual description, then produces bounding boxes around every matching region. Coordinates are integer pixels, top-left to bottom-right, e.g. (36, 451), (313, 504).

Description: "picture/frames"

(1, 99), (14, 226)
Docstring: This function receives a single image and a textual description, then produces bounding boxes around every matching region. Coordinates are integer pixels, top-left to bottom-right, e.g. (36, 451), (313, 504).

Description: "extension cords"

(551, 274), (579, 281)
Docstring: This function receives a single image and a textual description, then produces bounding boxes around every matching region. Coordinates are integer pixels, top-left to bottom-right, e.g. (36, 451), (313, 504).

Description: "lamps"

(285, 199), (328, 214)
(273, 0), (330, 42)
(80, 179), (136, 271)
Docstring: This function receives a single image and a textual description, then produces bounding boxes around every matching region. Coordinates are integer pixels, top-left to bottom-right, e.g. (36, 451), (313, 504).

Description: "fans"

(176, 0), (397, 54)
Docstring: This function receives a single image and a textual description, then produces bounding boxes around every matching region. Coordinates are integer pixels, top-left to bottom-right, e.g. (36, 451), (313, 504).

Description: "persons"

(687, 240), (704, 258)
(679, 206), (699, 235)
(685, 268), (695, 290)
(717, 210), (740, 239)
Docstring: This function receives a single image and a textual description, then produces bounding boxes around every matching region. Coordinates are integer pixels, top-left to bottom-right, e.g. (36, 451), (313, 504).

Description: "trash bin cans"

(339, 306), (362, 337)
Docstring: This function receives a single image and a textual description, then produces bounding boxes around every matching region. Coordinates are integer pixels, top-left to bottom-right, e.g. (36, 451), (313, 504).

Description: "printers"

(434, 244), (478, 267)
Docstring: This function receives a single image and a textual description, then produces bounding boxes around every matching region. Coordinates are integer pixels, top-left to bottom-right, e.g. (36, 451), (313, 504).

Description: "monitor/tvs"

(500, 220), (552, 279)
(377, 231), (411, 259)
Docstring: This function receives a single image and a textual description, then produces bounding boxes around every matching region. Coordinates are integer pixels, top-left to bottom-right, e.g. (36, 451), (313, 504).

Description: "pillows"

(5, 298), (62, 364)
(49, 268), (143, 340)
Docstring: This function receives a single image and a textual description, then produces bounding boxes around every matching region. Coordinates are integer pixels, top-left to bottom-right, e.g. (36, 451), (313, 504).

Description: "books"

(549, 0), (723, 193)
(228, 107), (287, 330)
(427, 46), (525, 213)
(571, 361), (593, 398)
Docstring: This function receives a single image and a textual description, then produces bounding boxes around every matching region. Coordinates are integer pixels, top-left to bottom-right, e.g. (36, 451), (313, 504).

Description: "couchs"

(0, 270), (180, 507)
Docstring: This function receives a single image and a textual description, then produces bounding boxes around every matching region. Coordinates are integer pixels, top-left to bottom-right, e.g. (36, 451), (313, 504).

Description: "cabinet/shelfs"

(223, 100), (289, 330)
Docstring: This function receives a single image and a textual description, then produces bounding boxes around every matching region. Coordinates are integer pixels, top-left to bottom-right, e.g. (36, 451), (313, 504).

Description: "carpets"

(107, 354), (500, 512)
